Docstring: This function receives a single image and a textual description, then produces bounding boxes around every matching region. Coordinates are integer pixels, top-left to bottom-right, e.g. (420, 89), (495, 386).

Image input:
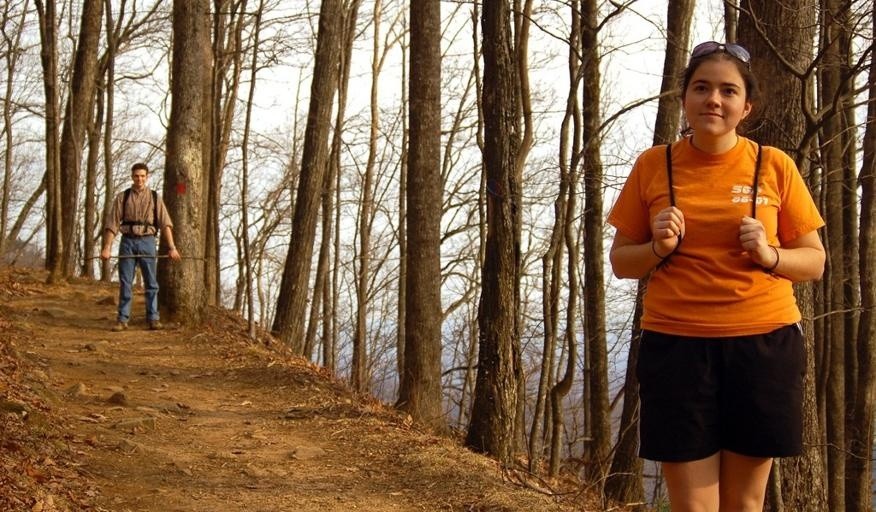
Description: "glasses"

(692, 41), (752, 68)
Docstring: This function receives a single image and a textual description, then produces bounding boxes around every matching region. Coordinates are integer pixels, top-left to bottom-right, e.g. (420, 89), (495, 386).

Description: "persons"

(607, 50), (826, 512)
(100, 164), (182, 332)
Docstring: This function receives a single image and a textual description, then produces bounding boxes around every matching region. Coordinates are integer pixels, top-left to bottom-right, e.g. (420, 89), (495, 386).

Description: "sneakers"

(111, 321), (129, 332)
(146, 319), (163, 329)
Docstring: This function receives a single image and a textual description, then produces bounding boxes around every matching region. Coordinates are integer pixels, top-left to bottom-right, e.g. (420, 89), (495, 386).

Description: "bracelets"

(652, 239), (668, 259)
(761, 245), (779, 271)
